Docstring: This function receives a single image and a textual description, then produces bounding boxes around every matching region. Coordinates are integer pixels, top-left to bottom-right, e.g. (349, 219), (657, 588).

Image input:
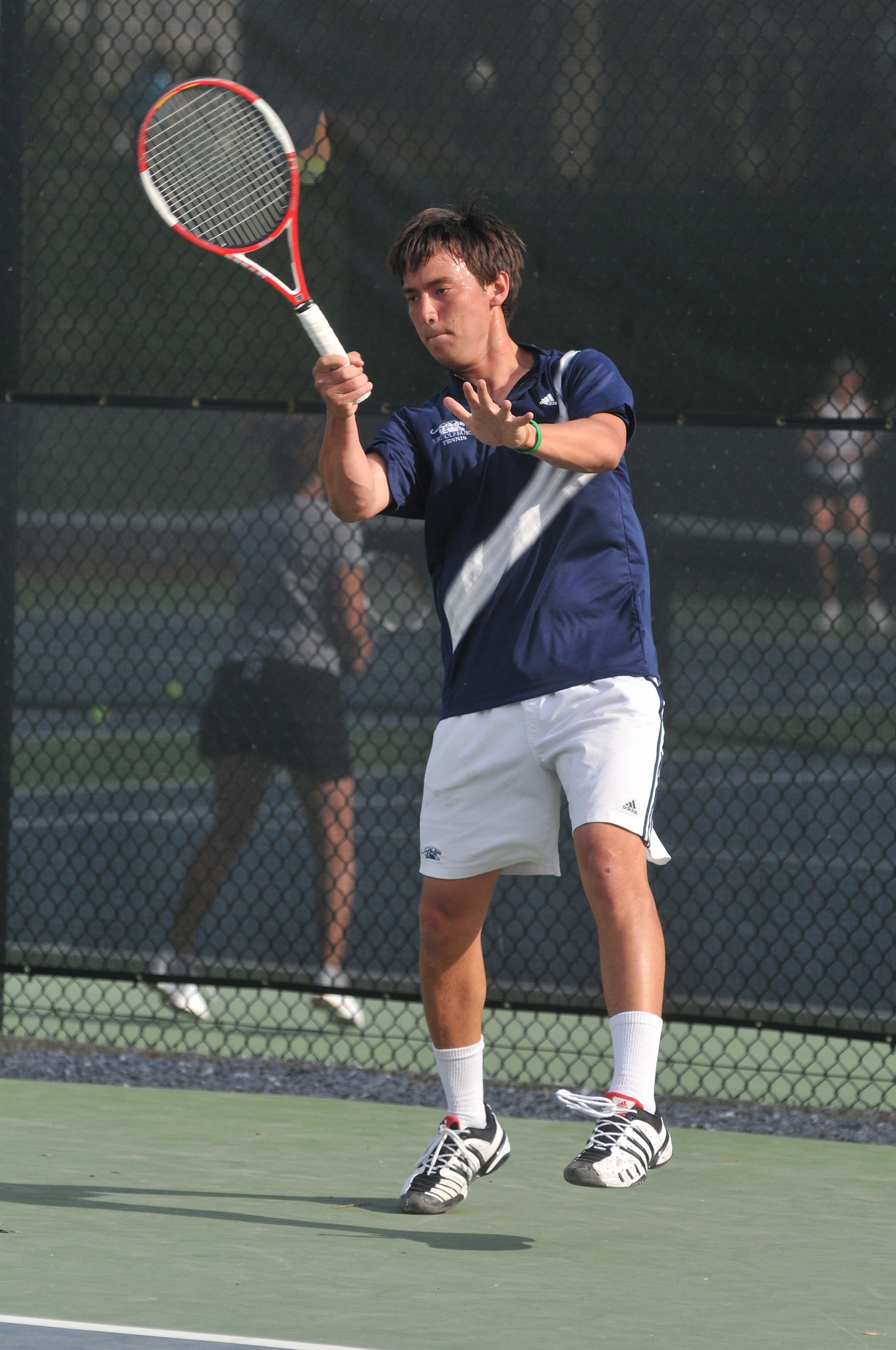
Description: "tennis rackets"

(341, 550), (436, 674)
(135, 76), (373, 406)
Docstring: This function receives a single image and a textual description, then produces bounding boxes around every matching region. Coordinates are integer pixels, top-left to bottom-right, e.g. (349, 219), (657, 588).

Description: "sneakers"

(313, 973), (365, 1030)
(399, 1102), (512, 1213)
(556, 1088), (674, 1190)
(147, 961), (210, 1021)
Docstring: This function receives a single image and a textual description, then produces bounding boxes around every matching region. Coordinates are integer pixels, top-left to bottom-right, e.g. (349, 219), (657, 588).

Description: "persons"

(797, 356), (893, 630)
(312, 210), (674, 1214)
(144, 418), (378, 1029)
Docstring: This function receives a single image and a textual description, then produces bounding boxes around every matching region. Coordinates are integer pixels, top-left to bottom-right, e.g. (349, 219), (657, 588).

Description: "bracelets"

(514, 420), (542, 455)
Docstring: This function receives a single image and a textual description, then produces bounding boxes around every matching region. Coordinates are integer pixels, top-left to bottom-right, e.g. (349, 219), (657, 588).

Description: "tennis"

(165, 679), (183, 699)
(88, 704), (111, 726)
